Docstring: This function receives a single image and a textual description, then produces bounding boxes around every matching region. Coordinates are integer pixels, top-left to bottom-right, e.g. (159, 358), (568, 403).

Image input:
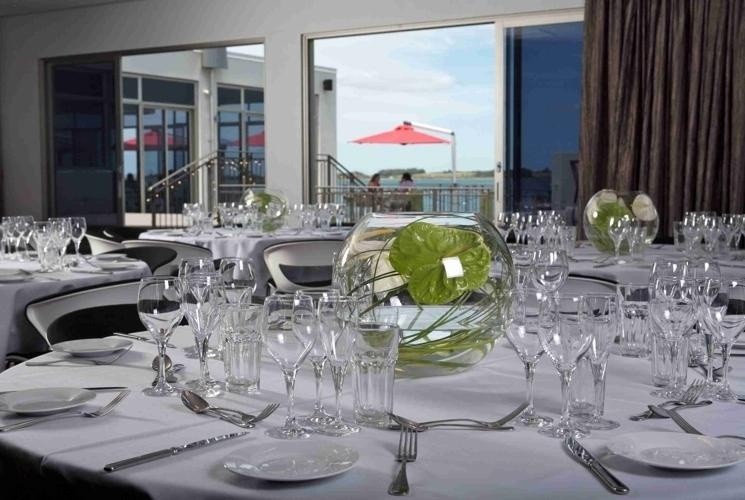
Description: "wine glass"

(182, 200), (347, 235)
(137, 256), (359, 434)
(0, 215), (87, 267)
(496, 209), (745, 437)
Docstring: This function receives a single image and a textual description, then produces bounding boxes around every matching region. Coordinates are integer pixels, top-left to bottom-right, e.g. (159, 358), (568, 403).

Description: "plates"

(226, 441), (358, 481)
(605, 430), (745, 472)
(0, 268), (31, 283)
(90, 260), (139, 270)
(0, 387), (94, 414)
(49, 336), (131, 358)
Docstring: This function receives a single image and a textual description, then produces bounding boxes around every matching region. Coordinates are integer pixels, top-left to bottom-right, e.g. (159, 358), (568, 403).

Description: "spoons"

(645, 401), (712, 419)
(386, 412), (514, 434)
(19, 269), (61, 281)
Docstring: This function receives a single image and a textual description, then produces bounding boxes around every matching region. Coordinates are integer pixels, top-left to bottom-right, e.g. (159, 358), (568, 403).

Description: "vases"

(581, 189), (659, 254)
(332, 212), (517, 375)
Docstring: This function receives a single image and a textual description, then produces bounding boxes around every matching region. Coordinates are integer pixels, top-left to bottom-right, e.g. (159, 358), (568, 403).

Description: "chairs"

(559, 274), (617, 307)
(83, 232), (124, 255)
(262, 238), (345, 298)
(46, 300), (187, 341)
(706, 283), (745, 341)
(25, 271), (187, 349)
(109, 247), (179, 277)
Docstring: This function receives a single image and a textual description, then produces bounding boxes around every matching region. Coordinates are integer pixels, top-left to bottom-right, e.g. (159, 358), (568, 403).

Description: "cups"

(349, 304), (401, 431)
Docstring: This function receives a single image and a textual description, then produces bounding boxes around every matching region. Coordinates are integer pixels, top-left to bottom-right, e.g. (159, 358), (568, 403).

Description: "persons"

(394, 172), (418, 193)
(367, 173), (382, 191)
(126, 173), (135, 184)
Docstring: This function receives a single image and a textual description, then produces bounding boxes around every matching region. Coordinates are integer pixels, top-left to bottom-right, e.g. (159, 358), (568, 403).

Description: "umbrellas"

(340, 121), (460, 187)
(124, 126), (200, 154)
(234, 130), (270, 147)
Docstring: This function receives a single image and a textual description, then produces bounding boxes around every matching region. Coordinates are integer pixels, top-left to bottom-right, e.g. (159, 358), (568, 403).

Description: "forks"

(386, 422), (418, 496)
(632, 378), (706, 422)
(26, 349), (128, 366)
(417, 402), (530, 426)
(666, 407), (745, 441)
(1, 389), (129, 433)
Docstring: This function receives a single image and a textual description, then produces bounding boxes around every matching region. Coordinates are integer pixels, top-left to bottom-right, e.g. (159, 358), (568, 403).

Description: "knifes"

(104, 431), (248, 472)
(561, 433), (628, 494)
(112, 332), (177, 350)
(69, 268), (111, 277)
